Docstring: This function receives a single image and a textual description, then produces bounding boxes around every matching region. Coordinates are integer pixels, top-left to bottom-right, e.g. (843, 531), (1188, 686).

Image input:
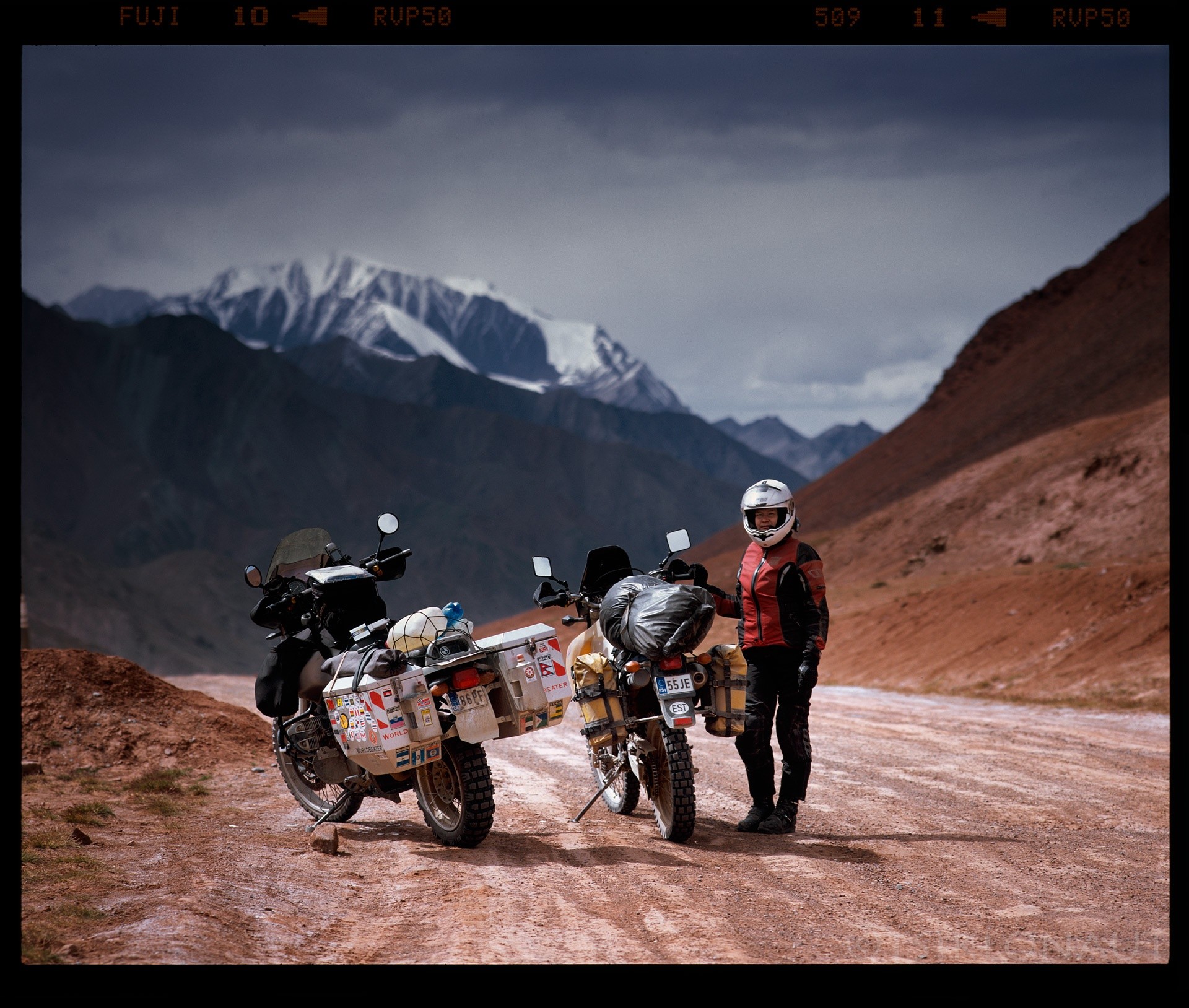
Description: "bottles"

(517, 654), (532, 667)
(437, 602), (464, 638)
(442, 617), (475, 636)
(385, 606), (447, 652)
(416, 682), (427, 696)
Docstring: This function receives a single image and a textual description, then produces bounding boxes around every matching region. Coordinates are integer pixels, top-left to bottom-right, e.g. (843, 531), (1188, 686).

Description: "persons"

(688, 479), (829, 834)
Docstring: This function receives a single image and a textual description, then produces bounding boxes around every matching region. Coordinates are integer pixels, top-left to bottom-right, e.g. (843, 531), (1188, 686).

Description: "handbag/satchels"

(255, 637), (313, 716)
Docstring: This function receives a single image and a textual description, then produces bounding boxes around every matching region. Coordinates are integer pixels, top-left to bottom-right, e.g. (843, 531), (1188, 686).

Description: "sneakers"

(759, 801), (798, 833)
(737, 796), (775, 832)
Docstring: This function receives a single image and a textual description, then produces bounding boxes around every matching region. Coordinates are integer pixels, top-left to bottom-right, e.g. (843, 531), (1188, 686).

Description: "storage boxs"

(322, 661), (442, 776)
(469, 623), (572, 740)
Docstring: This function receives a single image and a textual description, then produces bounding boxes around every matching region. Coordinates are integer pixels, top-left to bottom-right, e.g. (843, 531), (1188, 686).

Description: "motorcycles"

(530, 529), (711, 842)
(245, 511), (502, 849)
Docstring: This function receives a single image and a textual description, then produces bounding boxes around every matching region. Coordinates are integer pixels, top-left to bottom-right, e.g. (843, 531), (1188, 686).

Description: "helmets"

(740, 478), (796, 547)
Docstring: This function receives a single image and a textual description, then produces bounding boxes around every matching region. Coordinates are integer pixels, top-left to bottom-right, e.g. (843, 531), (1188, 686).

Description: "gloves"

(689, 563), (709, 589)
(797, 651), (820, 692)
